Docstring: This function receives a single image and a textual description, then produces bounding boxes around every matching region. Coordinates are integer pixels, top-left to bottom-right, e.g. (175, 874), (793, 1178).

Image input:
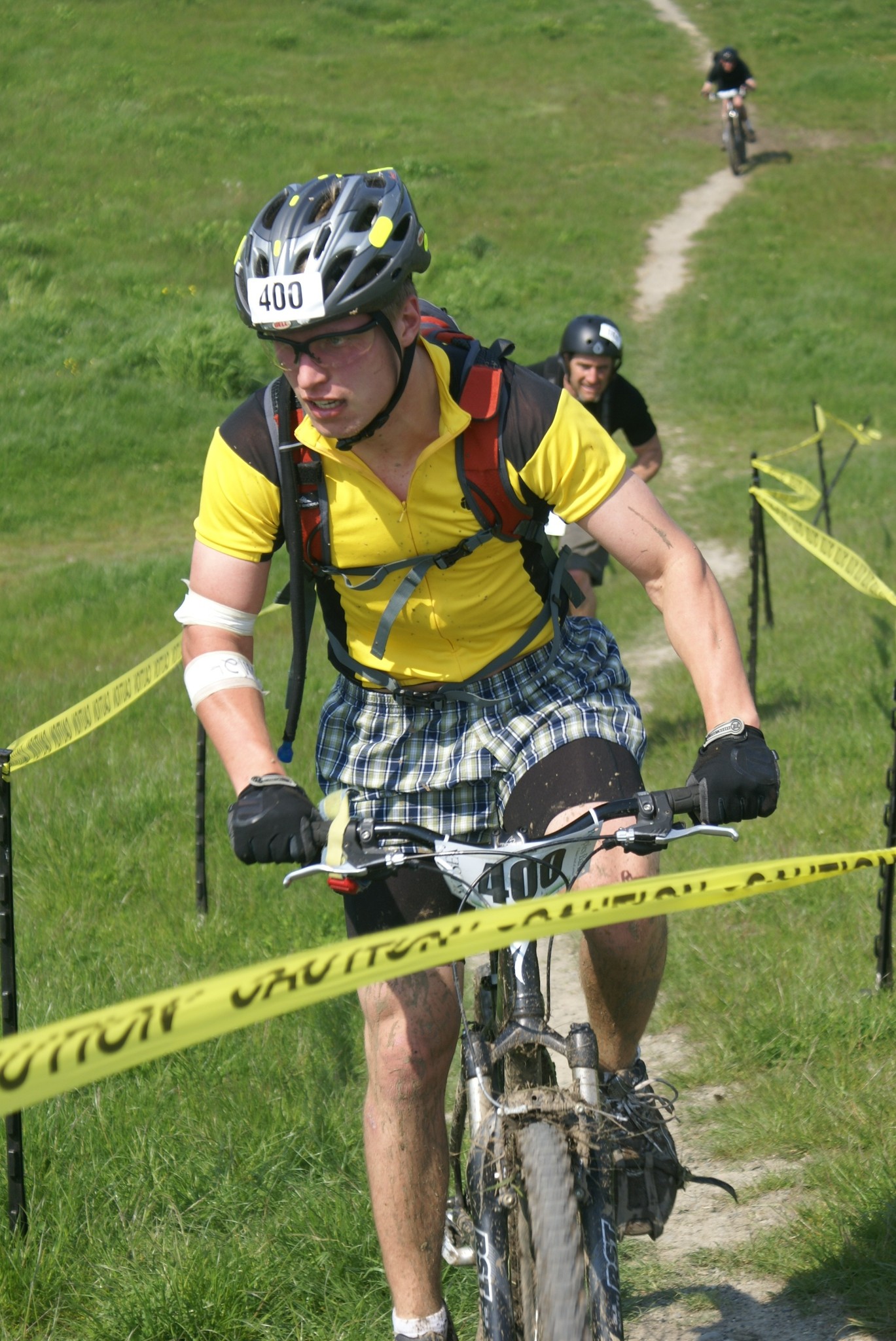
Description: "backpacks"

(261, 295), (585, 763)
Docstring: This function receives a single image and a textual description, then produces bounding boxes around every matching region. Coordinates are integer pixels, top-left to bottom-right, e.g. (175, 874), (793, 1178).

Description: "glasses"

(256, 314), (393, 371)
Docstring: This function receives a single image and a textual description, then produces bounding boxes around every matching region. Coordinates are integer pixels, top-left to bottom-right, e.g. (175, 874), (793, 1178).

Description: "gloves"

(227, 773), (331, 869)
(685, 717), (781, 827)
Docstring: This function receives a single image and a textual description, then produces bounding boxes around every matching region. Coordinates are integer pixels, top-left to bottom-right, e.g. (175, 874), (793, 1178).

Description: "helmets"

(233, 166), (431, 335)
(557, 314), (623, 370)
(717, 46), (739, 65)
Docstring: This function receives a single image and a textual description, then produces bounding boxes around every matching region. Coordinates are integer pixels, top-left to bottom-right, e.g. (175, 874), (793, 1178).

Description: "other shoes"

(744, 129), (756, 143)
(720, 138), (730, 152)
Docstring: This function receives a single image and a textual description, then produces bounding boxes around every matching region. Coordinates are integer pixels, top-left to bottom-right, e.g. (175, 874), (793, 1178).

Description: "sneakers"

(393, 1301), (457, 1341)
(591, 1058), (685, 1240)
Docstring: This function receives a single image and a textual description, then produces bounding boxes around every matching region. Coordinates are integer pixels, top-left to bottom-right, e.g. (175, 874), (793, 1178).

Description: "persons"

(175, 169), (784, 1341)
(523, 312), (665, 621)
(700, 47), (758, 152)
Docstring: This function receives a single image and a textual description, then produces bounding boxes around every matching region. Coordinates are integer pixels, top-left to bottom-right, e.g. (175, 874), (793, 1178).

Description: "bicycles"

(706, 81), (755, 171)
(278, 755), (754, 1341)
